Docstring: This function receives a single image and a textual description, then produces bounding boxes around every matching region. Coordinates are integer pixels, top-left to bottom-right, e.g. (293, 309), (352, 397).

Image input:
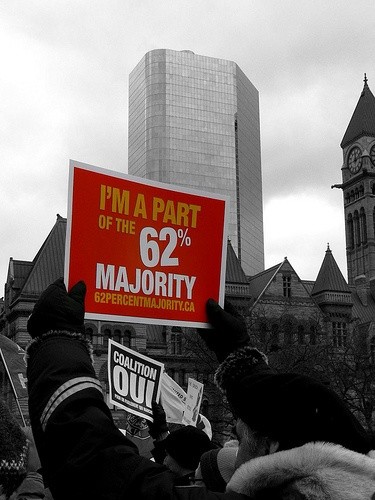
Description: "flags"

(0, 333), (28, 400)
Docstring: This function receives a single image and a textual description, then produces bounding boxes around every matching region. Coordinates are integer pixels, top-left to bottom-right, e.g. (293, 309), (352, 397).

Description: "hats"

(15, 471), (46, 496)
(199, 439), (239, 492)
(158, 426), (216, 463)
(228, 374), (370, 454)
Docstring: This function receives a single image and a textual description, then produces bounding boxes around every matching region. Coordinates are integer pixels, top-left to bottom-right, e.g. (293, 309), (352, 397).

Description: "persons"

(26, 276), (375, 499)
(0, 391), (239, 500)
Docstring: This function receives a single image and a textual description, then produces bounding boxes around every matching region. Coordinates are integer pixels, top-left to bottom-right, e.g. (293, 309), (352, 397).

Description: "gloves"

(27, 277), (87, 339)
(196, 298), (251, 363)
(147, 401), (169, 440)
